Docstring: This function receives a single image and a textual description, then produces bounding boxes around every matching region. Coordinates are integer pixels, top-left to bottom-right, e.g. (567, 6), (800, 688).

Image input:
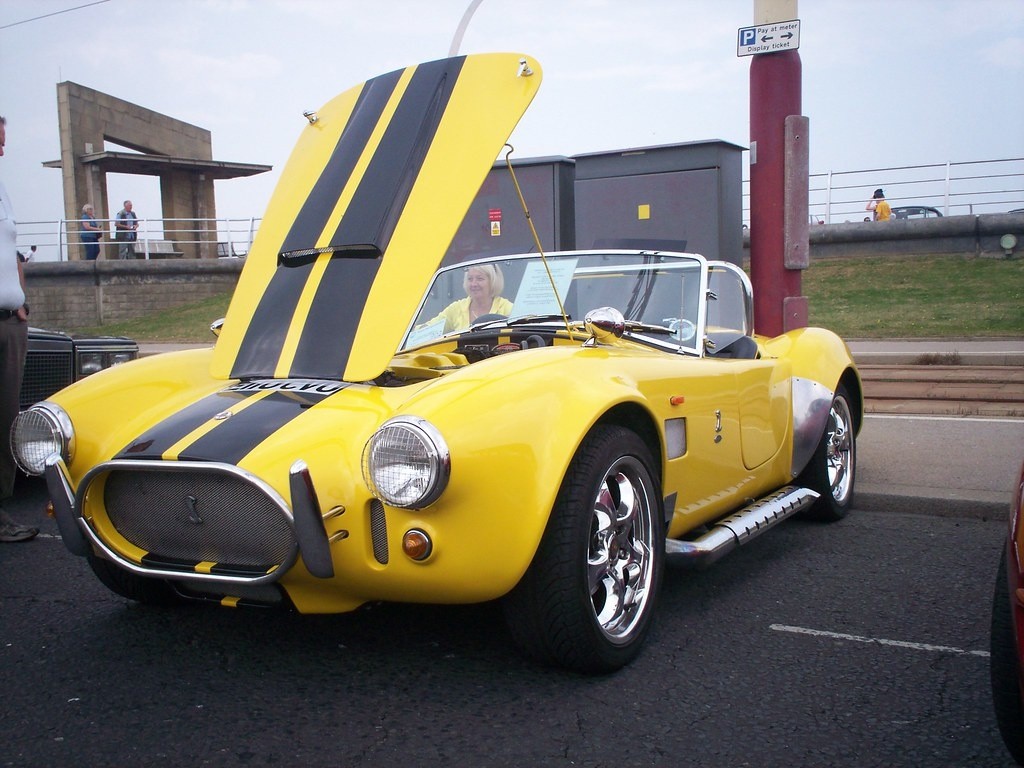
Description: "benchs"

(135, 239), (184, 259)
(217, 242), (246, 258)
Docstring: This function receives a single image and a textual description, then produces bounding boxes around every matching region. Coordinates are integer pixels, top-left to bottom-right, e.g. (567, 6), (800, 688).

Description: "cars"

(888, 206), (944, 219)
(10, 327), (140, 500)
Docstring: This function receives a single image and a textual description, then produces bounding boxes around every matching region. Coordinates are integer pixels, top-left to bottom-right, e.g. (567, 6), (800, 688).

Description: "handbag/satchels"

(97, 233), (102, 238)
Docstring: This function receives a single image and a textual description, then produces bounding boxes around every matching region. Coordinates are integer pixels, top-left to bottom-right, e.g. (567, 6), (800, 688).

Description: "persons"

(80, 203), (104, 261)
(113, 199), (139, 259)
(409, 262), (514, 339)
(863, 189), (891, 223)
(0, 118), (40, 543)
(25, 244), (36, 263)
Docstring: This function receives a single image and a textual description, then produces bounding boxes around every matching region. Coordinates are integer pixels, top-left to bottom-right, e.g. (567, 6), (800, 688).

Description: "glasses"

(866, 219), (870, 222)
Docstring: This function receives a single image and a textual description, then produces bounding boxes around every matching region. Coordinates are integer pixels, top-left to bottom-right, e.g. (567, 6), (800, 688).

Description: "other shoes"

(0, 506), (40, 542)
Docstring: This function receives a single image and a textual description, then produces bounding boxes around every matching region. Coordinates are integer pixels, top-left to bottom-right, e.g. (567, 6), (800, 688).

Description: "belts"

(0, 309), (17, 320)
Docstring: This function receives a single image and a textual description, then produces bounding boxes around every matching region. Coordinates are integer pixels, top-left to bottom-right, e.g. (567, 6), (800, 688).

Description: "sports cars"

(7, 50), (864, 678)
